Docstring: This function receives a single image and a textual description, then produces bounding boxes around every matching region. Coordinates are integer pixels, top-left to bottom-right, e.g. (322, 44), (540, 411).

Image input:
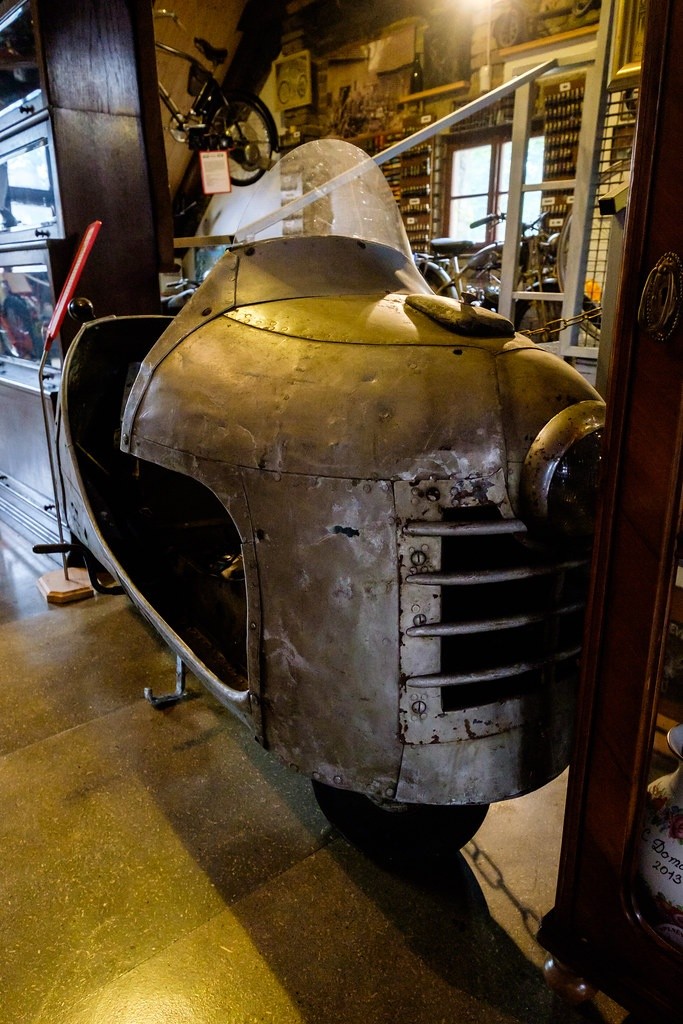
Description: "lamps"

(36, 219), (104, 603)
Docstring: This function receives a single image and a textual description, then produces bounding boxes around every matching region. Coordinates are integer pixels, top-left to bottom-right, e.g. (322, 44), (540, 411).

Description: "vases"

(638, 723), (683, 949)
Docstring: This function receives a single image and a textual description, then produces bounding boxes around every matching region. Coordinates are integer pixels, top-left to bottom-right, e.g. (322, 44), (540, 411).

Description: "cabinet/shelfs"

(0, 0), (176, 574)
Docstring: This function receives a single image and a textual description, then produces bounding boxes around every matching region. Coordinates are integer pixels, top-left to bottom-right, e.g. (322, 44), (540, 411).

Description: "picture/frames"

(272, 49), (313, 112)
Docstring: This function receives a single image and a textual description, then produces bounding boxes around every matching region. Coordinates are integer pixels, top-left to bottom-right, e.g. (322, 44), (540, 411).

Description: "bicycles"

(415, 208), (602, 347)
(153, 9), (282, 187)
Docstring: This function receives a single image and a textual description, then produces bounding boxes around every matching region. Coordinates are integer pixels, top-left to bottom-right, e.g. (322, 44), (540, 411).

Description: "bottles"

(410, 54), (422, 94)
(630, 723), (683, 954)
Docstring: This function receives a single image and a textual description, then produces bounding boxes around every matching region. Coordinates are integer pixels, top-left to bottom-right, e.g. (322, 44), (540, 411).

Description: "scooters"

(33, 138), (605, 857)
